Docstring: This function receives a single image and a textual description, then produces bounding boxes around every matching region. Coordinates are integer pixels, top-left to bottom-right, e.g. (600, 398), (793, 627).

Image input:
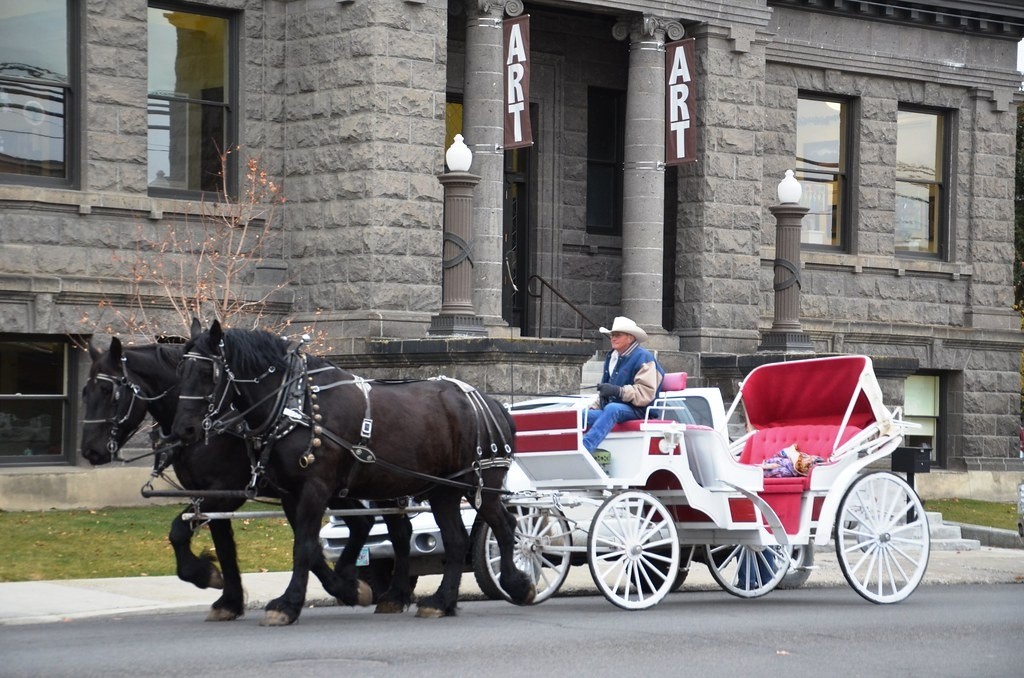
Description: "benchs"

(582, 373), (689, 478)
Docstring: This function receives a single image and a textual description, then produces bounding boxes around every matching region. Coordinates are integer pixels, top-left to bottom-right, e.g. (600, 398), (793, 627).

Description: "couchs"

(727, 425), (862, 535)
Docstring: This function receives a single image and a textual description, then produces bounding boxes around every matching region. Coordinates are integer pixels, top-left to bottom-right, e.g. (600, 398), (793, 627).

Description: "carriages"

(79, 317), (932, 628)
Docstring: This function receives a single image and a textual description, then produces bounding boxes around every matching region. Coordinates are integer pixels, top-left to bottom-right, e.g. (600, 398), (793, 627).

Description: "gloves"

(596, 382), (621, 398)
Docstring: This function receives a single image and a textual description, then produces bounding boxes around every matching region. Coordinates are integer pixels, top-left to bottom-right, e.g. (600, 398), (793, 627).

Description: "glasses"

(609, 333), (630, 339)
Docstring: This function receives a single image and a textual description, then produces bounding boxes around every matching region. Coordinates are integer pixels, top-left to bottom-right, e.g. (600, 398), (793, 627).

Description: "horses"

(80, 318), (536, 627)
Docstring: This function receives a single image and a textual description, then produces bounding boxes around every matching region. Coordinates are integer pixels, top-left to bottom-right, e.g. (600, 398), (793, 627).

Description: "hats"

(598, 316), (649, 343)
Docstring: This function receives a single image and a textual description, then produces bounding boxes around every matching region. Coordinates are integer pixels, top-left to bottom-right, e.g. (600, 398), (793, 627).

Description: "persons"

(582, 315), (667, 452)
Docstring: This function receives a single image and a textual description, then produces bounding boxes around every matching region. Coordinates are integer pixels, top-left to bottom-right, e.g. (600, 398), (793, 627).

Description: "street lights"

(425, 133), (492, 337)
(758, 168), (817, 350)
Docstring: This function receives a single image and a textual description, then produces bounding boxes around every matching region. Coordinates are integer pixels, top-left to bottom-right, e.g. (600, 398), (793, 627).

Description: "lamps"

(777, 169), (802, 202)
(446, 133), (473, 171)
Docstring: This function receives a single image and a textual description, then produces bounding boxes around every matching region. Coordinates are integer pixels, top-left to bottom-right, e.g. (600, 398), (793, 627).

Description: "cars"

(316, 386), (814, 606)
